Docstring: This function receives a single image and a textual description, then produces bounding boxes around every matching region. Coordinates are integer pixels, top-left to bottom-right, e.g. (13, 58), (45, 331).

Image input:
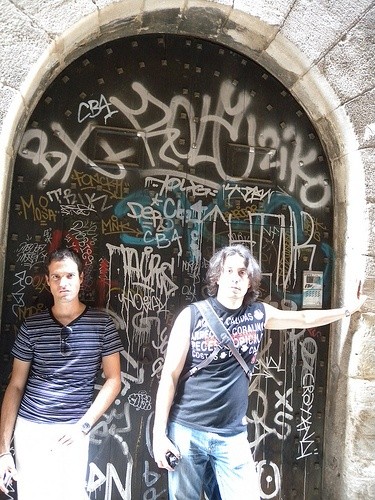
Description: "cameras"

(165, 452), (179, 469)
